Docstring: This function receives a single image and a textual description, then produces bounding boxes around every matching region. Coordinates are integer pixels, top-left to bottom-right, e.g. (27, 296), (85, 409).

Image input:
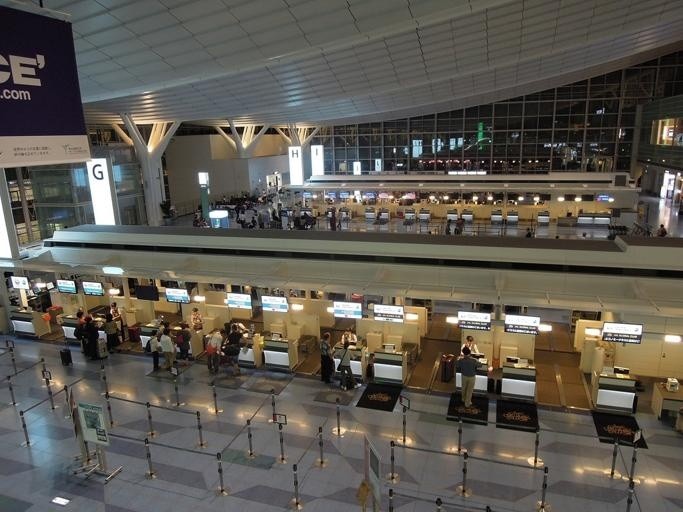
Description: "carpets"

(356, 383), (403, 412)
(446, 393), (488, 426)
(313, 382), (359, 405)
(209, 368), (255, 389)
(496, 400), (540, 433)
(590, 410), (646, 448)
(248, 372), (296, 396)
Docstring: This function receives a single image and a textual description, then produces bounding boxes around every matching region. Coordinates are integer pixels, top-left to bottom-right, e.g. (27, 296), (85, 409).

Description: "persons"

(580, 232), (587, 239)
(333, 341), (362, 391)
(657, 224), (667, 236)
(457, 345), (483, 408)
(320, 332), (334, 384)
(75, 301), (251, 374)
(337, 209), (343, 228)
(525, 227), (531, 238)
(341, 327), (358, 345)
(446, 218), (466, 235)
(222, 188), (301, 230)
(556, 235), (560, 239)
(461, 335), (479, 356)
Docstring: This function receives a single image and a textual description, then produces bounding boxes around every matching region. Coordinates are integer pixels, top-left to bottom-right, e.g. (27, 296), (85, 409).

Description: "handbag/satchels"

(206, 344), (216, 354)
(336, 364), (341, 371)
(73, 324), (84, 341)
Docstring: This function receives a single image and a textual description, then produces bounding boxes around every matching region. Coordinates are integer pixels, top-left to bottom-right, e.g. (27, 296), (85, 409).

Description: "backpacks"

(145, 338), (153, 352)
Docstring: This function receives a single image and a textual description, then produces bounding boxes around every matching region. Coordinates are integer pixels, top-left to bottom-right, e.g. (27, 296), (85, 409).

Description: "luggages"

(96, 338), (108, 358)
(439, 353), (454, 383)
(60, 341), (72, 366)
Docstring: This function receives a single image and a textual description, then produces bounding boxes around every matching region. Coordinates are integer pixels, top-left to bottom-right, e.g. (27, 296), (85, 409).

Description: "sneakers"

(353, 384), (361, 389)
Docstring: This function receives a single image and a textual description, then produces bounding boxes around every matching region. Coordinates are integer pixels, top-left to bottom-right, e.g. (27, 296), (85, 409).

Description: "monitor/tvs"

(82, 281), (105, 296)
(373, 304), (404, 323)
(11, 276), (29, 289)
(226, 292), (253, 310)
(261, 295), (288, 313)
(602, 322), (643, 344)
(166, 288), (190, 304)
(458, 310), (491, 331)
(57, 280), (77, 294)
(334, 301), (362, 320)
(505, 314), (540, 336)
(135, 285), (159, 301)
